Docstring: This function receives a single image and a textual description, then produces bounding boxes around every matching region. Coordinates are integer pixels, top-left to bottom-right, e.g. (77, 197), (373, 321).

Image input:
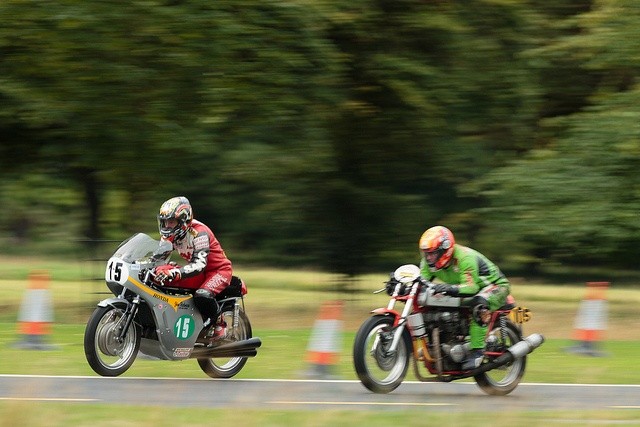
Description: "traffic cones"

(6, 269), (60, 351)
(563, 282), (610, 355)
(295, 301), (343, 379)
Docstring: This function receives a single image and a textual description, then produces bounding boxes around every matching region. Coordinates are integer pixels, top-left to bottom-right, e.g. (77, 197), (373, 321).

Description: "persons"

(136, 196), (232, 341)
(387, 225), (511, 370)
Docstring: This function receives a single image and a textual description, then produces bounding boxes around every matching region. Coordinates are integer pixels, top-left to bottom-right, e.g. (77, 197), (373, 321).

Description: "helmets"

(158, 196), (193, 242)
(419, 226), (455, 274)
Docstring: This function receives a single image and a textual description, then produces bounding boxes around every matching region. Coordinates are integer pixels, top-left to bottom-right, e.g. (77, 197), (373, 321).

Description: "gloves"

(154, 265), (182, 285)
(431, 282), (459, 298)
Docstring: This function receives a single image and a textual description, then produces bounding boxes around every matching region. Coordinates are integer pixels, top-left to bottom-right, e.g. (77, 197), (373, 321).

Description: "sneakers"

(206, 320), (228, 343)
(462, 347), (488, 371)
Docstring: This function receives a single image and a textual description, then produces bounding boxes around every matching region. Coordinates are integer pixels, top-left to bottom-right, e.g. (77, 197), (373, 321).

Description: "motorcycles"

(83, 232), (261, 378)
(354, 264), (544, 395)
